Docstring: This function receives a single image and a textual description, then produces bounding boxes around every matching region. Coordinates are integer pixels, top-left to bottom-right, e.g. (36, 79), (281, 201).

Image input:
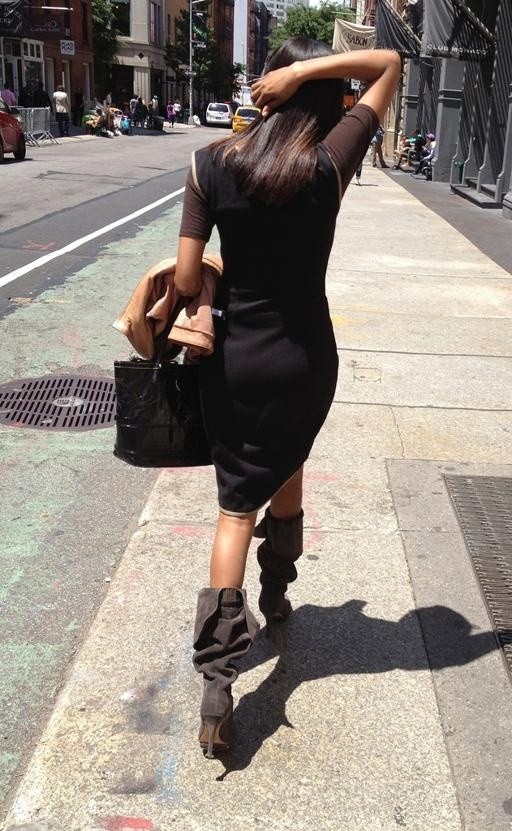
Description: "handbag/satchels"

(113, 291), (215, 468)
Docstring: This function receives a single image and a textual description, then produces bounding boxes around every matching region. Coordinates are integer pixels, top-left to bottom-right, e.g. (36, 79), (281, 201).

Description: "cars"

(0, 95), (26, 164)
(232, 105), (261, 134)
(205, 102), (234, 129)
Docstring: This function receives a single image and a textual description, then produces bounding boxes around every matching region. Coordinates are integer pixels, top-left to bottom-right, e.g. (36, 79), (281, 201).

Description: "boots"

(193, 585), (259, 759)
(252, 506), (304, 645)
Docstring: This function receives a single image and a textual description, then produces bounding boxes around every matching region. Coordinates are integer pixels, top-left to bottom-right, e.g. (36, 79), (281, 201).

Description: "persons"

(53, 78), (186, 139)
(1, 83), (17, 106)
(18, 86), (34, 108)
(168, 32), (404, 764)
(370, 120), (439, 180)
(33, 84), (54, 112)
